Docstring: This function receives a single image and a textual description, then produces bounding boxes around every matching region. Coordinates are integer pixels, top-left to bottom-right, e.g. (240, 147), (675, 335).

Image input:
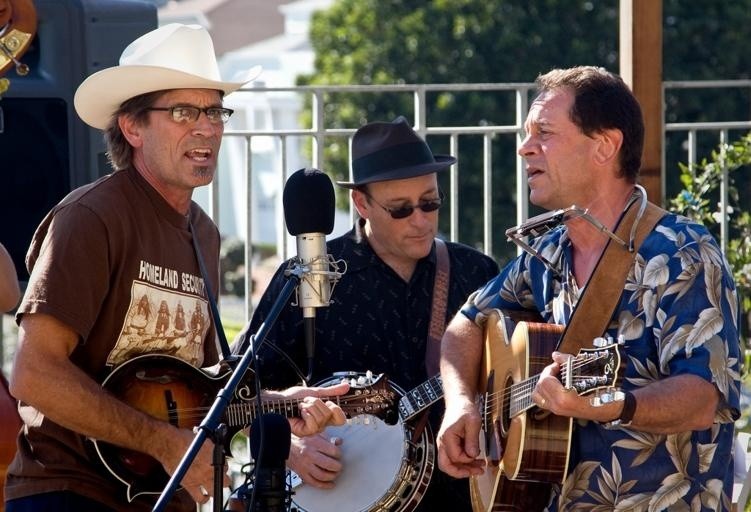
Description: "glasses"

(145, 105), (234, 125)
(364, 187), (445, 219)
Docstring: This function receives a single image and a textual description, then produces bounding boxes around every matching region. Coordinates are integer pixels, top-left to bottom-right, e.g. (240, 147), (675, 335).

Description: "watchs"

(598, 388), (636, 432)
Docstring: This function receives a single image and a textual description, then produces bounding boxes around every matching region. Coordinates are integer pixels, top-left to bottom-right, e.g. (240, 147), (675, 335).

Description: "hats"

(335, 115), (457, 189)
(73, 22), (263, 131)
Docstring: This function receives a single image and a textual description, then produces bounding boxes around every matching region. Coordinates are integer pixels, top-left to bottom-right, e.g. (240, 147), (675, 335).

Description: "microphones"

(280, 167), (337, 317)
(250, 413), (291, 512)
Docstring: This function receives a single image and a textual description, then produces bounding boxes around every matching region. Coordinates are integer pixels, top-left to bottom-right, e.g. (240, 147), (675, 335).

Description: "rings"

(199, 485), (209, 498)
(330, 437), (337, 444)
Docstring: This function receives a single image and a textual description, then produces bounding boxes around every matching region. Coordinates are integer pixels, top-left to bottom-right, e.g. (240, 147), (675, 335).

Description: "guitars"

(92, 352), (398, 505)
(469, 308), (626, 511)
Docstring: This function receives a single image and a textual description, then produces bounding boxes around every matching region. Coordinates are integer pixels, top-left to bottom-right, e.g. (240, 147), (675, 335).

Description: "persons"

(2, 22), (350, 512)
(435, 65), (742, 512)
(232, 116), (503, 512)
(0, 241), (30, 512)
